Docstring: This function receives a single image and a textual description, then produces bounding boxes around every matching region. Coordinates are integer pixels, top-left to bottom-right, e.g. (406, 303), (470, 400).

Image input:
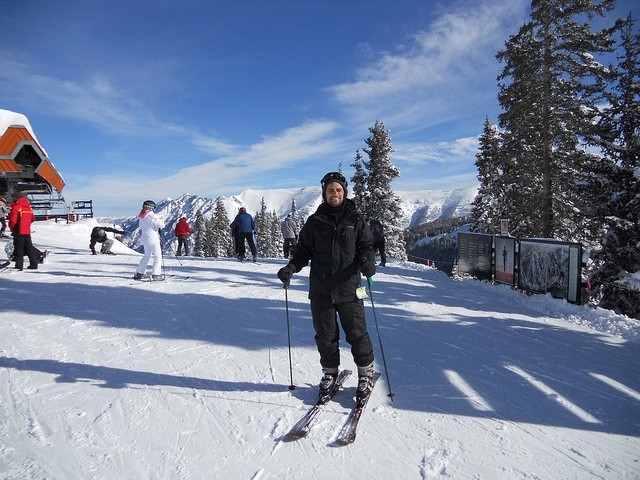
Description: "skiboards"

(285, 369), (381, 446)
(237, 257), (261, 265)
(128, 274), (190, 282)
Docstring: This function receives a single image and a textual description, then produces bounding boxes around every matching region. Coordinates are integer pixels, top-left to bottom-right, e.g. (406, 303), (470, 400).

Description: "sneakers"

(357, 374), (372, 398)
(151, 275), (163, 281)
(319, 374), (338, 395)
(133, 273), (151, 280)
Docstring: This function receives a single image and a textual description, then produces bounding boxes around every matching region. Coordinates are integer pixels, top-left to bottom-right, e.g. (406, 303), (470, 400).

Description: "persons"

(5, 236), (50, 261)
(277, 172), (377, 403)
(365, 219), (387, 271)
(13, 191), (40, 270)
(281, 213), (296, 258)
(89, 226), (128, 257)
(0, 197), (11, 239)
(133, 201), (165, 282)
(229, 207), (259, 263)
(174, 216), (192, 256)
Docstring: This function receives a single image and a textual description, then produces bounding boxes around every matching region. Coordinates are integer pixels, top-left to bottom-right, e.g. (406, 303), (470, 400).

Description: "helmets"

(321, 172), (348, 192)
(143, 200), (156, 212)
(239, 207), (247, 212)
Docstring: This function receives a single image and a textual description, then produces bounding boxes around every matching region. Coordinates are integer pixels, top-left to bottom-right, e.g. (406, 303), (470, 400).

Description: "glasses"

(322, 174), (346, 181)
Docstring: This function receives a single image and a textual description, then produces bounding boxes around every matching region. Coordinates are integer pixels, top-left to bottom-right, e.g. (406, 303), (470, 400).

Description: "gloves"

(360, 258), (376, 278)
(277, 263), (296, 284)
